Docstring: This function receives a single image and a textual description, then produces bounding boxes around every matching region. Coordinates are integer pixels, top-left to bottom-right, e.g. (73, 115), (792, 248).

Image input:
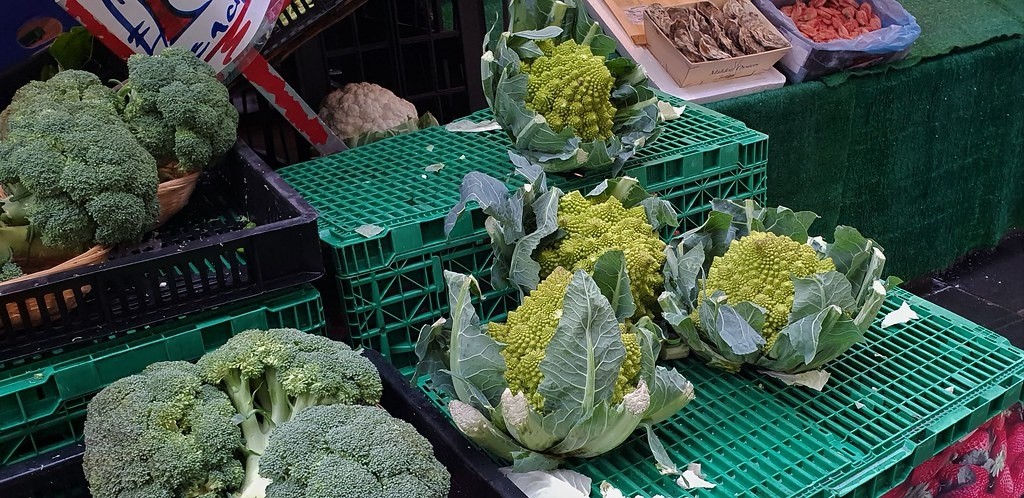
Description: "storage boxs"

(643, 0), (794, 86)
(274, 86), (769, 377)
(0, 135), (325, 371)
(751, 0), (922, 85)
(409, 277), (1024, 498)
(0, 283), (328, 470)
(0, 350), (528, 498)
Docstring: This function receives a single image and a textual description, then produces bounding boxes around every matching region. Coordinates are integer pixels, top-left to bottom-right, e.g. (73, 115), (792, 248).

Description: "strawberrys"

(877, 401), (1024, 498)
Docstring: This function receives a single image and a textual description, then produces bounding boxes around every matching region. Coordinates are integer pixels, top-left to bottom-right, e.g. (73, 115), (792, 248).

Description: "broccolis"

(412, 166), (903, 450)
(315, 82), (418, 147)
(82, 328), (453, 498)
(0, 46), (239, 279)
(481, 0), (658, 171)
